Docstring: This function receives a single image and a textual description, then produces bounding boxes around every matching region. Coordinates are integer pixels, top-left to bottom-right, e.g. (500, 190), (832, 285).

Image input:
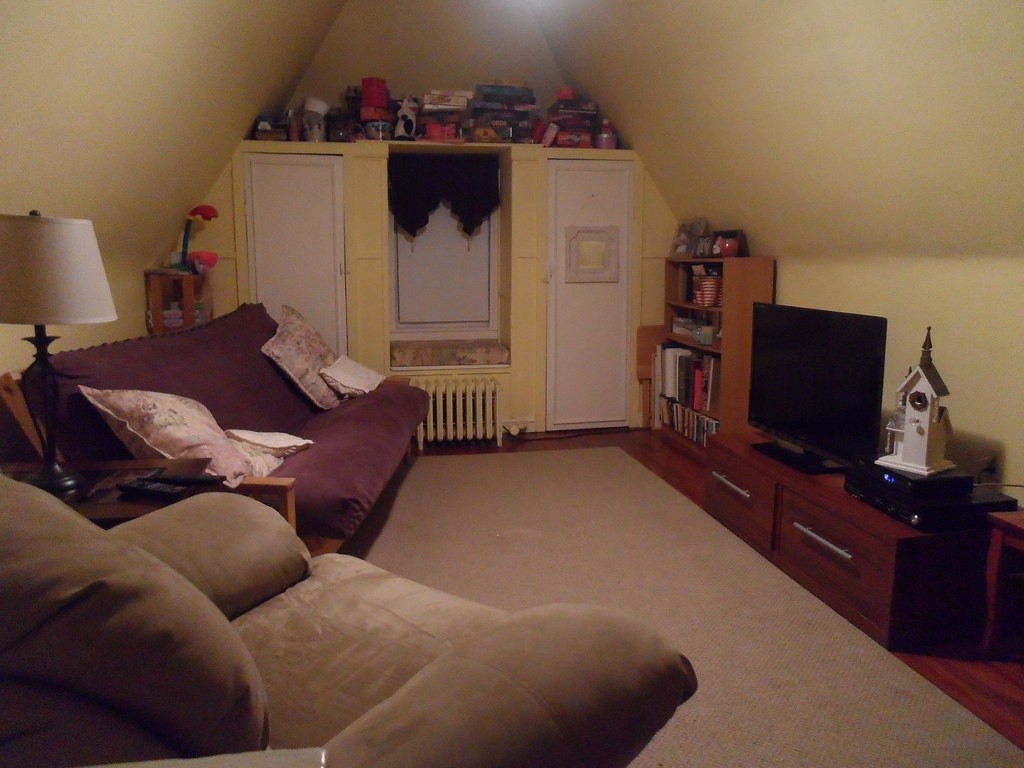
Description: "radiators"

(406, 377), (519, 451)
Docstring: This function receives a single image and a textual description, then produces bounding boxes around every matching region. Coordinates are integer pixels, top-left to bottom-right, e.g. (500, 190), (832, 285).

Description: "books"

(662, 345), (719, 447)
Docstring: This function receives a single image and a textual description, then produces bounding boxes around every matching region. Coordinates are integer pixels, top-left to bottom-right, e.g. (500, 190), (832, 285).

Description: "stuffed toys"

(165, 249), (219, 277)
(393, 94), (419, 140)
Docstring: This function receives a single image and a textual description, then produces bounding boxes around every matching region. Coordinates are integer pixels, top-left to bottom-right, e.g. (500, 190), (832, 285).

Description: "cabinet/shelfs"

(659, 256), (777, 466)
(702, 430), (1021, 649)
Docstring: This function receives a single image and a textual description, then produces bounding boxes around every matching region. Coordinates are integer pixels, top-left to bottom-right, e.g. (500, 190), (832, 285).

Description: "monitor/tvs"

(748, 300), (887, 475)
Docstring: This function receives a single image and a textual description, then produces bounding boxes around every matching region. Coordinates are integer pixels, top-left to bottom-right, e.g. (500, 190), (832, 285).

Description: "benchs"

(390, 338), (509, 368)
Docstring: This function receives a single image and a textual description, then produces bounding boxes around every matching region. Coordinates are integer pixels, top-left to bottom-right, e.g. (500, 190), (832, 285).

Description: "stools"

(146, 268), (211, 332)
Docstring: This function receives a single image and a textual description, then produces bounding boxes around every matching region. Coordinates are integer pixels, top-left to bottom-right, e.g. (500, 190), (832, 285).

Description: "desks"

(972, 510), (1022, 653)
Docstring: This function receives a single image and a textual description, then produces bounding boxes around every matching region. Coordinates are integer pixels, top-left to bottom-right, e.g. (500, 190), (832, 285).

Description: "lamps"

(169, 205), (219, 272)
(0, 207), (119, 503)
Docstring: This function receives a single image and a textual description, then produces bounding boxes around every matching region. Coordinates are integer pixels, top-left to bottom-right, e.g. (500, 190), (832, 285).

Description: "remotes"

(141, 474), (228, 485)
(116, 478), (188, 502)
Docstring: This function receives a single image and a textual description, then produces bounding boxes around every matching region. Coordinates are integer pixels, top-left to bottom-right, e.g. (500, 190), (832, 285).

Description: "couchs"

(0, 475), (696, 768)
(26, 302), (426, 560)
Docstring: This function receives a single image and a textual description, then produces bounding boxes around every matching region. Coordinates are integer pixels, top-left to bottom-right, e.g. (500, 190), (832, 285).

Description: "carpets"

(361, 450), (1023, 768)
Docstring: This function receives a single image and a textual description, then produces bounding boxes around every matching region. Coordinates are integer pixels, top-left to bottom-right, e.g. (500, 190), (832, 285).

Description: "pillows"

(260, 307), (343, 410)
(318, 356), (388, 395)
(221, 425), (315, 460)
(77, 382), (285, 487)
